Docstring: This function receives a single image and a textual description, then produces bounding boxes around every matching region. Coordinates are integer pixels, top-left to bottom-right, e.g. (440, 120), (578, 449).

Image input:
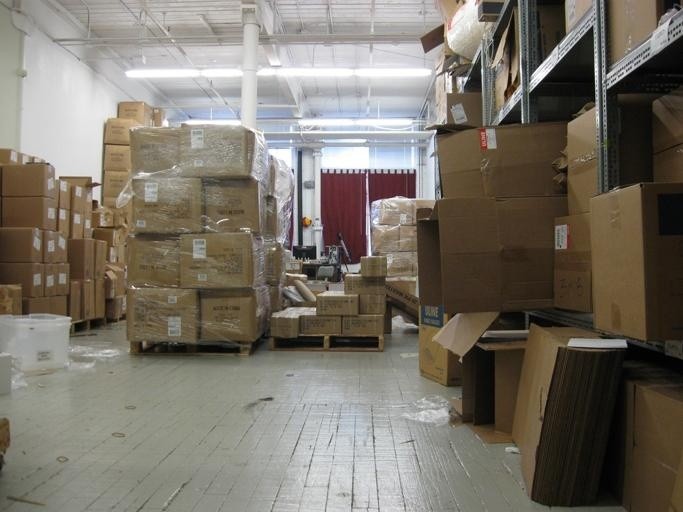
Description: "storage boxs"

(0, 148), (103, 397)
(102, 101), (294, 357)
(275, 198), (436, 354)
(416, 0), (683, 511)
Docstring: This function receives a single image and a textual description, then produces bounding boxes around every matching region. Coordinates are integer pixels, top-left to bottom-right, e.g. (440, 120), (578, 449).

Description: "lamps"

(122, 43), (435, 78)
(295, 100), (415, 130)
